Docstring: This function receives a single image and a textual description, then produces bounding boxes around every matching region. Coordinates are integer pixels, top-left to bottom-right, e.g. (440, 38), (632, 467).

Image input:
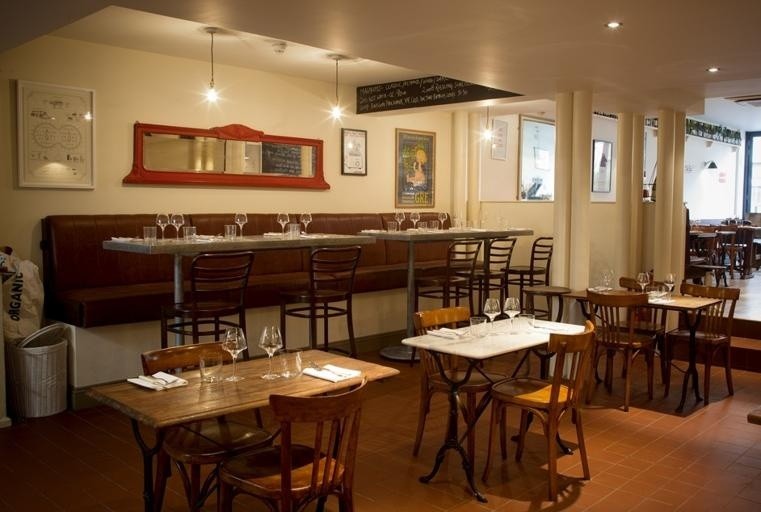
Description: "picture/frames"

(14, 77), (98, 192)
(517, 112), (556, 201)
(340, 126), (370, 177)
(490, 118), (510, 161)
(591, 137), (614, 195)
(394, 127), (440, 211)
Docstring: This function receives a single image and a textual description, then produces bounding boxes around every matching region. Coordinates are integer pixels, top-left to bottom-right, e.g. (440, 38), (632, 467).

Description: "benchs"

(39, 210), (485, 389)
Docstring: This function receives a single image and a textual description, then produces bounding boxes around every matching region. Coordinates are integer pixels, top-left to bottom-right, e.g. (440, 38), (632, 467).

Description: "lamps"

(201, 26), (221, 102)
(328, 55), (349, 120)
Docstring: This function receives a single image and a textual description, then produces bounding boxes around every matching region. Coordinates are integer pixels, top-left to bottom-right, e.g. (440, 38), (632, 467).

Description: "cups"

(181, 226), (197, 241)
(198, 350), (223, 381)
(417, 220), (440, 234)
(223, 224), (237, 239)
(469, 316), (487, 338)
(199, 382), (226, 409)
(518, 313), (534, 334)
(386, 221), (397, 232)
(280, 349), (303, 379)
(143, 226), (157, 242)
(289, 223), (301, 239)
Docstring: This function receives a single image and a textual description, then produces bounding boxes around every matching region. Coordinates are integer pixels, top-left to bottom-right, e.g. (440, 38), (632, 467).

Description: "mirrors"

(121, 119), (333, 194)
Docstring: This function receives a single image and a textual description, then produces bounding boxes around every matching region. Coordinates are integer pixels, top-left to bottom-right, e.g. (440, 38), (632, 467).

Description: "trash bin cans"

(11, 336), (68, 417)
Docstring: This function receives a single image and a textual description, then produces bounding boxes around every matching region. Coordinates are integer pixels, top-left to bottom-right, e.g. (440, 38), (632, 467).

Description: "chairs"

(584, 286), (656, 410)
(666, 279), (741, 404)
(218, 373), (371, 511)
(413, 303), (510, 483)
(142, 338), (277, 511)
(415, 238), (483, 328)
(488, 320), (597, 502)
(498, 235), (556, 323)
(159, 249), (258, 366)
(598, 277), (675, 399)
(279, 244), (363, 363)
(455, 236), (517, 319)
(685, 209), (761, 287)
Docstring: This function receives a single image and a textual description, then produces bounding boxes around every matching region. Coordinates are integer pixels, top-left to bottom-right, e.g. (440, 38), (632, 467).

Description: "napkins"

(125, 370), (189, 392)
(302, 364), (362, 382)
(425, 327), (472, 339)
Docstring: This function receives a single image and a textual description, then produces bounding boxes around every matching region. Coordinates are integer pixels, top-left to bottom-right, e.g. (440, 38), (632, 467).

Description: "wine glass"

(483, 298), (503, 336)
(394, 208), (421, 233)
(299, 209), (315, 236)
(234, 210), (249, 239)
(662, 271), (679, 303)
(276, 211), (290, 238)
(438, 210), (448, 230)
(256, 325), (283, 381)
(223, 327), (248, 382)
(155, 212), (186, 243)
(503, 296), (521, 334)
(600, 268), (615, 291)
(635, 272), (651, 294)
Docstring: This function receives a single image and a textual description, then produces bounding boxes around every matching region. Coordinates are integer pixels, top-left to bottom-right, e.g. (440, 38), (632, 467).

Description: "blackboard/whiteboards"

(260, 142), (302, 176)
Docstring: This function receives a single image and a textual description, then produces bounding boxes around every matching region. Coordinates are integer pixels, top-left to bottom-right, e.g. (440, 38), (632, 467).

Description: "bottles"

(643, 171), (650, 198)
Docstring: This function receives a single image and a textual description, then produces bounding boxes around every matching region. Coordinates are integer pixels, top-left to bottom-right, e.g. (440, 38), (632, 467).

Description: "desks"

(522, 284), (572, 323)
(400, 315), (591, 502)
(101, 232), (377, 377)
(359, 227), (537, 361)
(82, 347), (402, 510)
(559, 285), (723, 415)
